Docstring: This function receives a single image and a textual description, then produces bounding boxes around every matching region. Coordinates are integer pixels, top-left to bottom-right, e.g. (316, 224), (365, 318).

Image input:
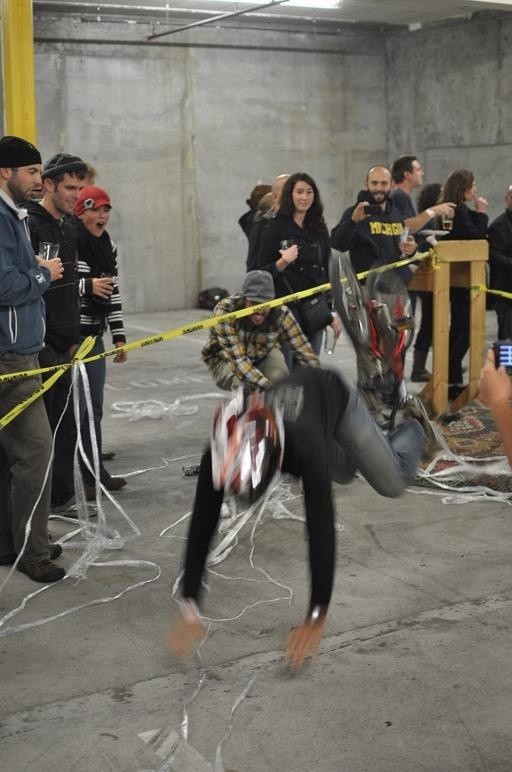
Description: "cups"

(92, 273), (117, 305)
(38, 241), (60, 268)
(442, 208), (454, 230)
(280, 238), (296, 266)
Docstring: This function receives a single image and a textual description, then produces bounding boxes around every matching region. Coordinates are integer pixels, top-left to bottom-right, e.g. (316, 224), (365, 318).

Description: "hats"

(41, 153), (86, 179)
(0, 136), (42, 168)
(241, 270), (275, 302)
(75, 185), (112, 215)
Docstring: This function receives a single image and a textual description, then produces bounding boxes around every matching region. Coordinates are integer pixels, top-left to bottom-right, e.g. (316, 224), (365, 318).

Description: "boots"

(412, 350), (430, 381)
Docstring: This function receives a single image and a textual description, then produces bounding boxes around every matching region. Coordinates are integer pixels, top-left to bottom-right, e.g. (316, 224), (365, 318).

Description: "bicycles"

(327, 251), (416, 436)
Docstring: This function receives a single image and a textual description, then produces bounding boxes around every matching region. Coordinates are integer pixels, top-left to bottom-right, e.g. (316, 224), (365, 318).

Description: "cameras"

(490, 340), (512, 376)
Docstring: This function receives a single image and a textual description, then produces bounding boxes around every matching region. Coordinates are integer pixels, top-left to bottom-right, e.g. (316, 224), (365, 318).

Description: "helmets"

(209, 402), (285, 498)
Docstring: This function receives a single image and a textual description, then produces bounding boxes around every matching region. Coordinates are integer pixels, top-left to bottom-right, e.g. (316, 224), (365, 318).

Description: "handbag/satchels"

(299, 292), (334, 335)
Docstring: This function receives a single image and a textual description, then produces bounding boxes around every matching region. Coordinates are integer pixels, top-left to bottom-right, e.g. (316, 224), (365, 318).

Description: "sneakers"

(0, 544), (62, 565)
(101, 478), (126, 490)
(403, 398), (438, 447)
(17, 559), (66, 582)
(51, 502), (97, 517)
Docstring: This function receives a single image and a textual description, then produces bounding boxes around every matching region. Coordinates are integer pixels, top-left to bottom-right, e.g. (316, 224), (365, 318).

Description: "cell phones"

(363, 206), (382, 215)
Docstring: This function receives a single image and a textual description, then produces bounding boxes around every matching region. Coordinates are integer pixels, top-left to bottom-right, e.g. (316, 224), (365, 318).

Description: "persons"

(238, 172), (342, 356)
(331, 166), (418, 408)
(388, 158), (512, 401)
(166, 366), (437, 674)
(200, 269), (320, 393)
(478, 347), (512, 468)
(19, 154), (128, 517)
(0, 135), (65, 586)
